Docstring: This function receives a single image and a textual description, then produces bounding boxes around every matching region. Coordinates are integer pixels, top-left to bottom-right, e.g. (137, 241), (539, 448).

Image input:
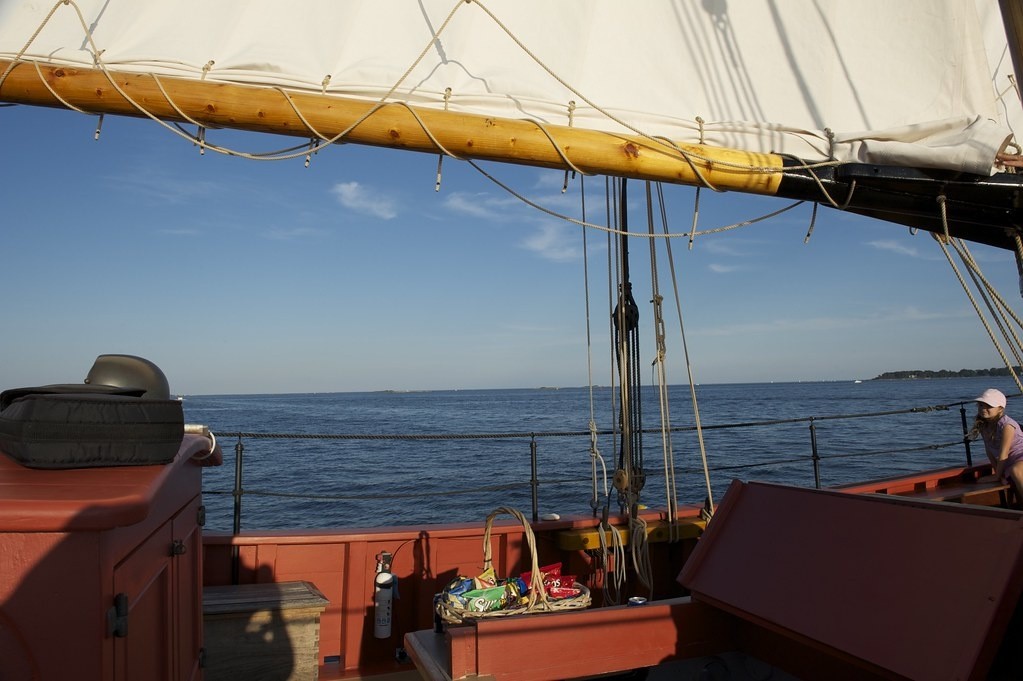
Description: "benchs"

(402, 596), (738, 681)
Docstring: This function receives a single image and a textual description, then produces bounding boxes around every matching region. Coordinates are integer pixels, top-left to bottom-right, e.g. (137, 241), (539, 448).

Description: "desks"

(199, 579), (331, 681)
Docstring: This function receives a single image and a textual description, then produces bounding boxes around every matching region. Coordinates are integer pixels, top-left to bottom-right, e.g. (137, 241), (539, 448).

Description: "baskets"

(435, 506), (592, 632)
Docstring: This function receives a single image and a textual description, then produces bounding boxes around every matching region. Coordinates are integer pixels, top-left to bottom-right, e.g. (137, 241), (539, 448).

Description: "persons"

(974, 388), (1023, 495)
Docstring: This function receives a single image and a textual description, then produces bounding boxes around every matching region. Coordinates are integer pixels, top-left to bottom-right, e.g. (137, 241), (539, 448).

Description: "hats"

(974, 388), (1007, 408)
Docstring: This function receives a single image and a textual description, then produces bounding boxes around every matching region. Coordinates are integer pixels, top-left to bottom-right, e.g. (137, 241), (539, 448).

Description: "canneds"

(627, 596), (648, 607)
